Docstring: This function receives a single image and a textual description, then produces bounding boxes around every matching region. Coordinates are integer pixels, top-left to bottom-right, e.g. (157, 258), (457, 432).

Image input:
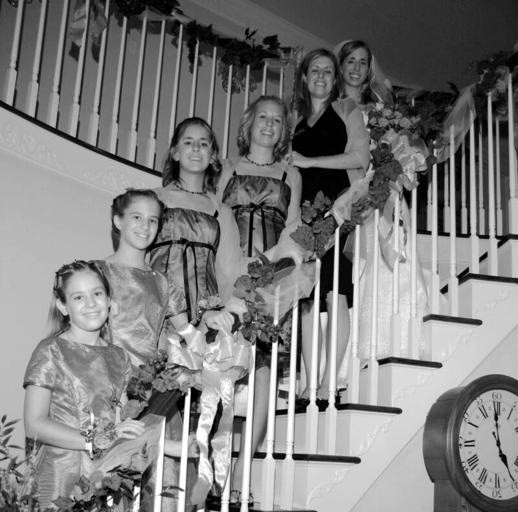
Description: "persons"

(335, 38), (424, 369)
(282, 47), (369, 413)
(211, 96), (301, 455)
(146, 116), (274, 512)
(98, 189), (235, 512)
(14, 260), (149, 512)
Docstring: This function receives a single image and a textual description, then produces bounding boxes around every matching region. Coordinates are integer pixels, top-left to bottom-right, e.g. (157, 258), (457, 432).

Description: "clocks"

(423, 372), (518, 511)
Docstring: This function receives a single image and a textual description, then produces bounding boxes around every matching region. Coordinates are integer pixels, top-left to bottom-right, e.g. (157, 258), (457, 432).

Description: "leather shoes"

(206, 495), (221, 510)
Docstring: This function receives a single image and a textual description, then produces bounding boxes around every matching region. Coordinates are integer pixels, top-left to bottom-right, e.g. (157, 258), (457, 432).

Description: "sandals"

(229, 489), (253, 509)
(295, 390), (340, 412)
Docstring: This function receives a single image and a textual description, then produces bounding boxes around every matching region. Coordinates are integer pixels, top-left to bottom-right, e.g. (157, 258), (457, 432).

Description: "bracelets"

(81, 423), (100, 458)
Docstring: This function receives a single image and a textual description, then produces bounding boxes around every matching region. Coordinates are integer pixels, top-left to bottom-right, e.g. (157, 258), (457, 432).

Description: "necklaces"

(244, 150), (278, 167)
(173, 180), (208, 195)
